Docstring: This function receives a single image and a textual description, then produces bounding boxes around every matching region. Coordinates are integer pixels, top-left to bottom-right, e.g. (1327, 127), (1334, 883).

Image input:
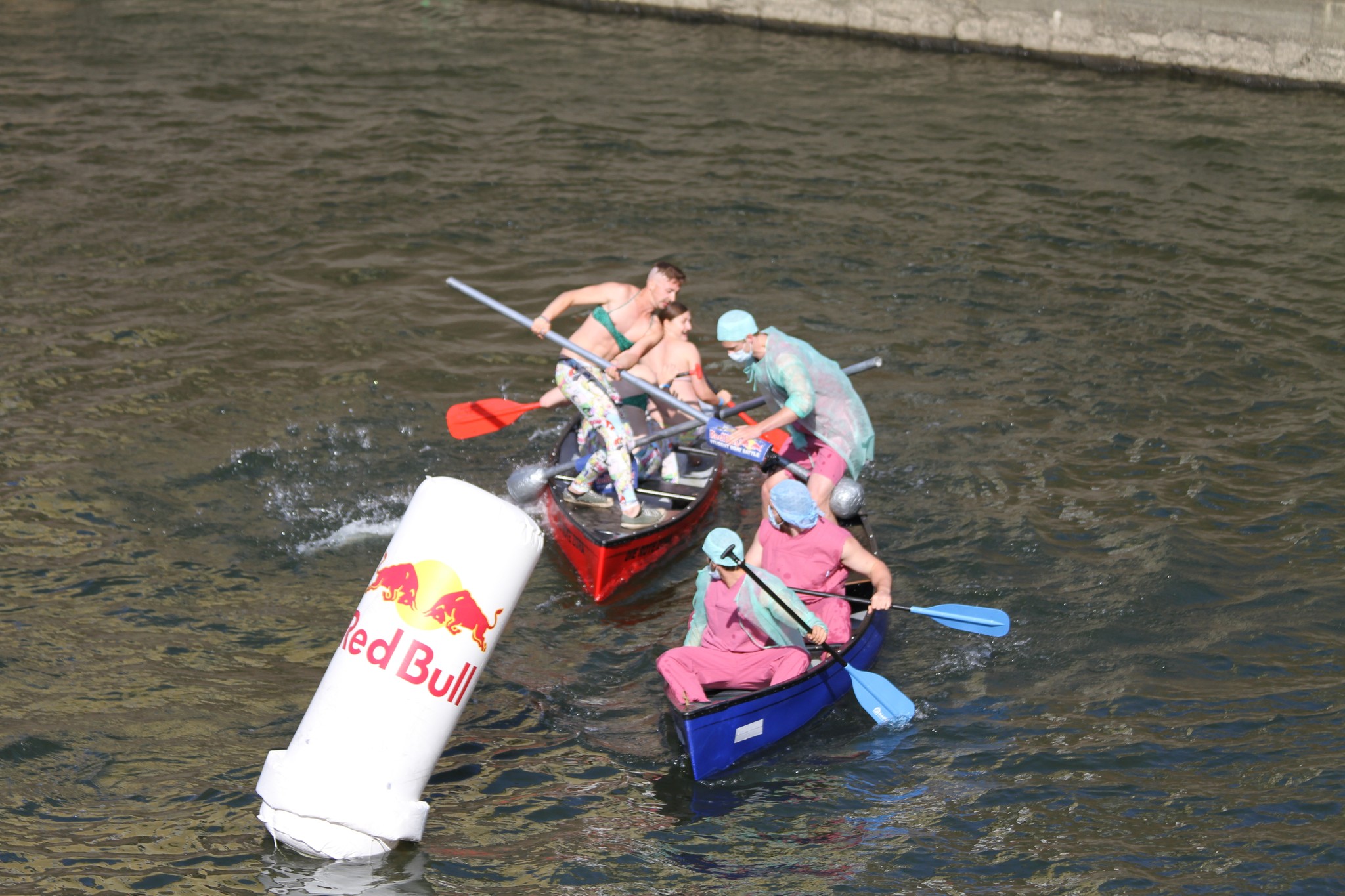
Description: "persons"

(745, 480), (892, 662)
(537, 302), (732, 484)
(531, 261), (686, 529)
(656, 528), (829, 705)
(717, 310), (876, 525)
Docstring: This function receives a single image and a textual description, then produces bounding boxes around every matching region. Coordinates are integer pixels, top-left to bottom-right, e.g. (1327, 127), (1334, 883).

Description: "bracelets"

(660, 383), (671, 389)
(718, 397), (724, 406)
(540, 315), (551, 323)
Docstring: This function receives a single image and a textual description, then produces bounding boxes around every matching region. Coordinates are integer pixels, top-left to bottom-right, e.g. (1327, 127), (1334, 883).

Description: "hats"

(769, 479), (824, 528)
(716, 309), (757, 341)
(702, 527), (743, 566)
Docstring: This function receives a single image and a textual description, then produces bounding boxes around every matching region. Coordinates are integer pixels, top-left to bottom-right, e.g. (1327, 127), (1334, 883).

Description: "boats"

(538, 368), (728, 604)
(665, 505), (891, 781)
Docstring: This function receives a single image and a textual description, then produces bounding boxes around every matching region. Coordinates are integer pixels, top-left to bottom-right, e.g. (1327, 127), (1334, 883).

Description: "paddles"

(446, 364), (704, 440)
(789, 582), (1012, 641)
(704, 377), (791, 453)
(724, 546), (915, 724)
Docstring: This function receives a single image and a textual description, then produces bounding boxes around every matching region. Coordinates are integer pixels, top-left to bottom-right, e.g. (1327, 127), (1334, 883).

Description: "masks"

(768, 505), (784, 530)
(708, 560), (720, 579)
(728, 342), (753, 363)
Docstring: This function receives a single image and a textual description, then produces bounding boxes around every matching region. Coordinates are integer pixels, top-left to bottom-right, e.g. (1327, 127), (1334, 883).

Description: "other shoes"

(620, 499), (667, 530)
(563, 485), (614, 508)
